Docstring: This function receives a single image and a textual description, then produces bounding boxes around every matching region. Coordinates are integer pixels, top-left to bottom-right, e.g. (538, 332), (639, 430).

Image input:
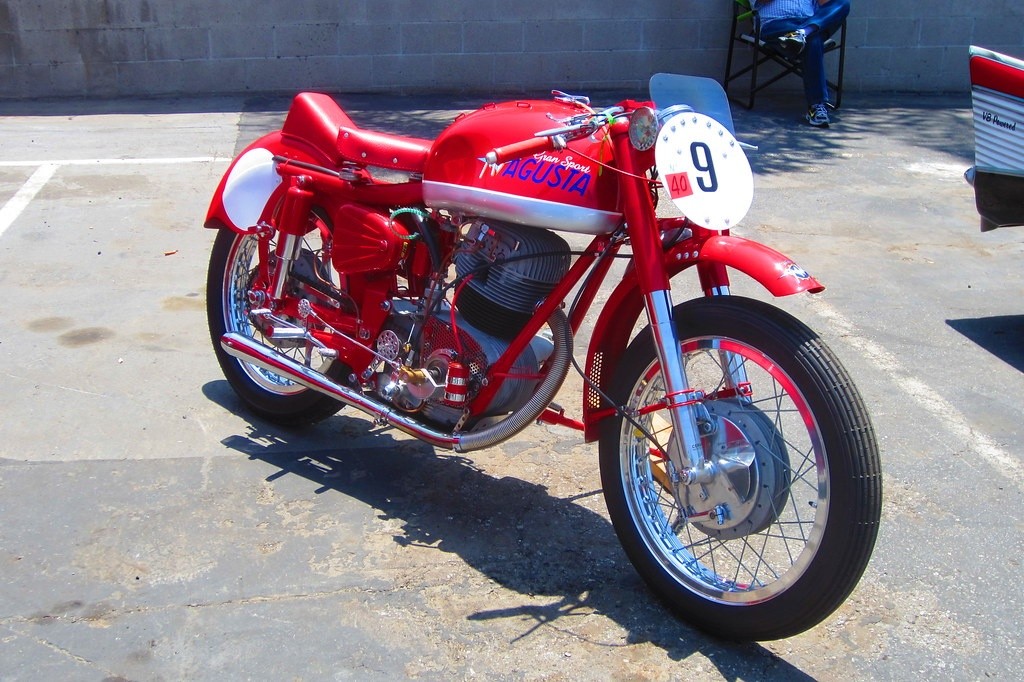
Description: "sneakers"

(804, 104), (829, 127)
(774, 33), (804, 57)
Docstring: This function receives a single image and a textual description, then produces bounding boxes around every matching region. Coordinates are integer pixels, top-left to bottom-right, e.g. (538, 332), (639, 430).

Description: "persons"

(749, 0), (851, 128)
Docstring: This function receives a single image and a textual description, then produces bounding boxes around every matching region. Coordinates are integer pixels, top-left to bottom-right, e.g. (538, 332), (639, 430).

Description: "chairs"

(723, 0), (847, 111)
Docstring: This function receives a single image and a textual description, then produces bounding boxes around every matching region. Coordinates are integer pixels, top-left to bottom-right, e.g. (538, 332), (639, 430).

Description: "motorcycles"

(203, 72), (885, 642)
